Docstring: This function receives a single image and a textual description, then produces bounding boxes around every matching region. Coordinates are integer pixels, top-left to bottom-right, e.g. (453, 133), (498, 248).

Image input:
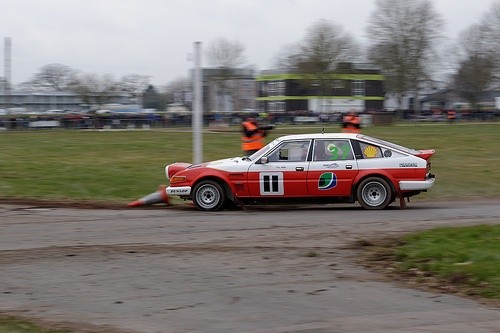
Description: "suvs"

(0, 103), (191, 130)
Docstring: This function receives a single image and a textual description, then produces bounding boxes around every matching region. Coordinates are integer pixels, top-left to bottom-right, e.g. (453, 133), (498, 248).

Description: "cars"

(165, 131), (437, 210)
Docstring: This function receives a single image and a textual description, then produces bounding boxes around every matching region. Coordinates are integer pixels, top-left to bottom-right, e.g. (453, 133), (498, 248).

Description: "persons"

(341, 109), (361, 133)
(240, 113), (273, 156)
(300, 143), (309, 161)
